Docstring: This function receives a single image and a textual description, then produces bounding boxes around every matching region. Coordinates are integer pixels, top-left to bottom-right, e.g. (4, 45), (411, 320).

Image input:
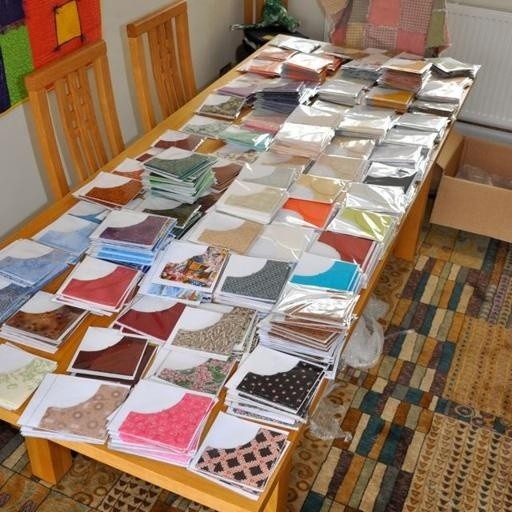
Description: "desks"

(0, 33), (486, 512)
(236, 27), (311, 66)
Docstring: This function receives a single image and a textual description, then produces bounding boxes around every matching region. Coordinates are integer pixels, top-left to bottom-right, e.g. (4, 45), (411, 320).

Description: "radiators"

(437, 1), (512, 134)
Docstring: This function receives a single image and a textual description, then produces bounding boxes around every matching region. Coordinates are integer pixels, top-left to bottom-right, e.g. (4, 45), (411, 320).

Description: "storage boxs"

(430, 128), (512, 245)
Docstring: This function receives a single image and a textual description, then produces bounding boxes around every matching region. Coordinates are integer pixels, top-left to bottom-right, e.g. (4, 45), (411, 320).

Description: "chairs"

(21, 38), (127, 201)
(125, 0), (199, 133)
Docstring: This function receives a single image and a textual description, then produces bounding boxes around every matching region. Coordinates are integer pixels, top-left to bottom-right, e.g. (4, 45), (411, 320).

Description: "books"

(1, 30), (484, 504)
